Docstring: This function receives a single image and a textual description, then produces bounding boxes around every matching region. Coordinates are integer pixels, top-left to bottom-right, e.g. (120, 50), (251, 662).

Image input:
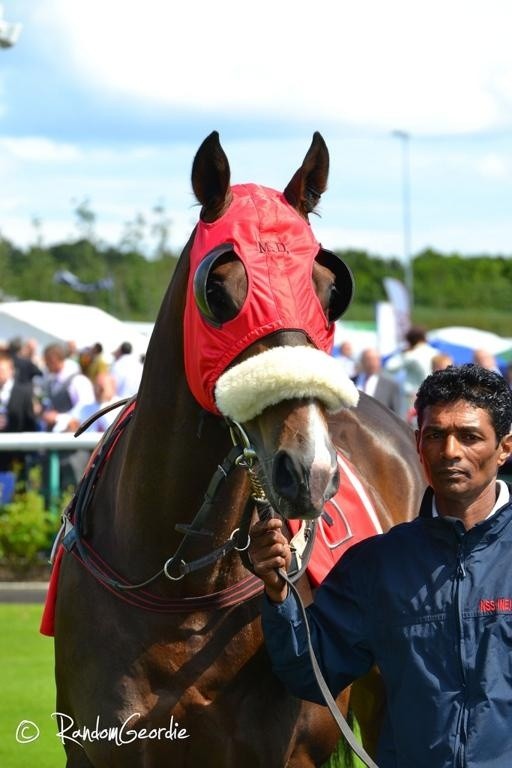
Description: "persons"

(0, 325), (512, 502)
(242, 364), (512, 768)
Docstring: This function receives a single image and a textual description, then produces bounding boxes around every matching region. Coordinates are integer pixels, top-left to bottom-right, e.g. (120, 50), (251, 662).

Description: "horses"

(54, 130), (426, 768)
